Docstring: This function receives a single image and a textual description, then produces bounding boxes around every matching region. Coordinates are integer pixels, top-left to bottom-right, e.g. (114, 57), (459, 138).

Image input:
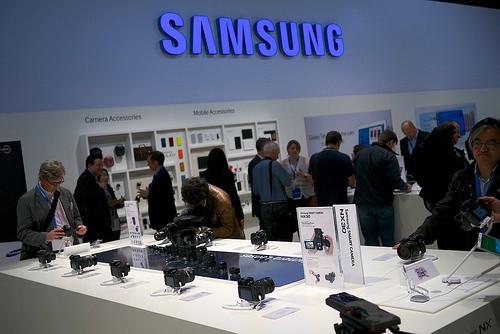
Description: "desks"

(0, 232), (500, 334)
(347, 179), (439, 251)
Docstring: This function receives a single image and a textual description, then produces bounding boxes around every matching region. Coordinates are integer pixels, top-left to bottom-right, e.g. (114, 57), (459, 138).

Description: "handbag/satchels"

(104, 155), (114, 168)
(20, 243), (35, 262)
(115, 144), (125, 156)
(271, 203), (298, 236)
(133, 144), (154, 161)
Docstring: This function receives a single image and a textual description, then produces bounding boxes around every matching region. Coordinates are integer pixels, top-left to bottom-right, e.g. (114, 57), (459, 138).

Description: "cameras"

(58, 225), (77, 237)
(163, 267), (195, 288)
(397, 232), (426, 260)
(458, 198), (493, 231)
(238, 276), (275, 305)
(325, 292), (401, 334)
(38, 250), (56, 264)
(69, 254), (97, 274)
(110, 259), (130, 278)
(250, 229), (268, 245)
(305, 228), (329, 251)
(325, 272), (335, 283)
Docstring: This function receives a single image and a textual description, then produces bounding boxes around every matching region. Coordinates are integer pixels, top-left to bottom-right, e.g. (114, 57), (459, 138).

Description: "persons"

(137, 151), (177, 232)
(308, 231), (333, 257)
(181, 176), (245, 239)
(204, 148), (245, 228)
(74, 153), (127, 243)
(17, 160), (87, 262)
(248, 116), (500, 252)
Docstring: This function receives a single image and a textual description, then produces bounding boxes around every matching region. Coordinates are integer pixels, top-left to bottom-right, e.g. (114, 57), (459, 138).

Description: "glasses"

(471, 139), (500, 149)
(46, 177), (64, 185)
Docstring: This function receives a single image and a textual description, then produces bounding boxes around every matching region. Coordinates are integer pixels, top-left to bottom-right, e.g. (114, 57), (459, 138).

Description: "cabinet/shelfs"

(83, 118), (284, 238)
